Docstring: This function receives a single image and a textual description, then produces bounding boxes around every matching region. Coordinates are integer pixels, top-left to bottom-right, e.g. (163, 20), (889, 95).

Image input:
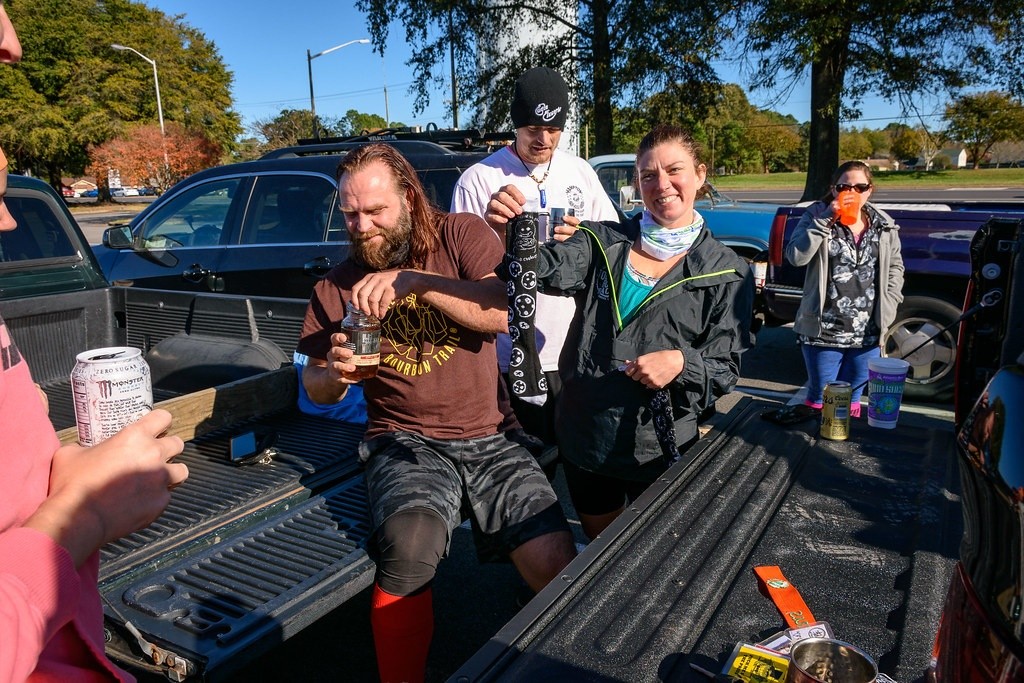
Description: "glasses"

(832, 182), (872, 193)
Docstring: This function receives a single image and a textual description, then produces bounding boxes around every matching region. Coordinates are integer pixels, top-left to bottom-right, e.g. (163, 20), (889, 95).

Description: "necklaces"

(514, 138), (552, 208)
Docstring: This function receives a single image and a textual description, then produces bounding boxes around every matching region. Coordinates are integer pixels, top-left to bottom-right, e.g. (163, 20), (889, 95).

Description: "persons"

(959, 391), (1024, 505)
(788, 161), (904, 418)
(297, 143), (577, 682)
(483, 124), (757, 542)
(0, 0), (188, 683)
(448, 66), (620, 618)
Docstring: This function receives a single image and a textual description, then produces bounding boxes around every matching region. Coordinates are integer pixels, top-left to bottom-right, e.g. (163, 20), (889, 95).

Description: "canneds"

(339, 300), (381, 381)
(69, 346), (153, 447)
(820, 380), (851, 442)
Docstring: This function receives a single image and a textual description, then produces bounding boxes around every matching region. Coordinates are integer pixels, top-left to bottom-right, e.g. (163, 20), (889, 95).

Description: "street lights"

(307, 39), (370, 138)
(111, 44), (170, 180)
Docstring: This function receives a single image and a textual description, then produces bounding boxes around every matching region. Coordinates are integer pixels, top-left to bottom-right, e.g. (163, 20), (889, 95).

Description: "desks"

(446, 393), (967, 682)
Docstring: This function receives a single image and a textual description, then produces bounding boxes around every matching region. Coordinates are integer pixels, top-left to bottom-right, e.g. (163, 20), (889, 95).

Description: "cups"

(867, 356), (910, 430)
(788, 638), (880, 683)
(837, 190), (862, 226)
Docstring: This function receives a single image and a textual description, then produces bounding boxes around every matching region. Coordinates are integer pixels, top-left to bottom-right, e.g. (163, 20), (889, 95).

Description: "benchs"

(97, 411), (559, 683)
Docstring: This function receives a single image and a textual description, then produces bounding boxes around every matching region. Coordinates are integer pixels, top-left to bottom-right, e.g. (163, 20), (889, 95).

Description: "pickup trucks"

(0, 173), (560, 683)
(764, 197), (1024, 401)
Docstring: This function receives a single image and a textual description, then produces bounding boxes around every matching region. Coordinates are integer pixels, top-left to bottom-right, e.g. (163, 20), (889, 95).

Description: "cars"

(586, 153), (785, 334)
(80, 188), (154, 197)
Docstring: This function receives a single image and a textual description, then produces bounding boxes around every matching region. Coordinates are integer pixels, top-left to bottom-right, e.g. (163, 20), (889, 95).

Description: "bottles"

(340, 298), (380, 382)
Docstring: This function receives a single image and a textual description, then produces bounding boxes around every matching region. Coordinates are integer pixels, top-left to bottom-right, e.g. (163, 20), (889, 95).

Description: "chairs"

(275, 189), (322, 240)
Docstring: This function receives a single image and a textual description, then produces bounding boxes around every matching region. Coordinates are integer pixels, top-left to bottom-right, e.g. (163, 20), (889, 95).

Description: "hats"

(510, 66), (568, 131)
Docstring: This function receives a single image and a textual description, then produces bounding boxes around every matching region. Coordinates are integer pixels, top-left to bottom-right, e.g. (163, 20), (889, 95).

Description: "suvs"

(90, 122), (517, 300)
